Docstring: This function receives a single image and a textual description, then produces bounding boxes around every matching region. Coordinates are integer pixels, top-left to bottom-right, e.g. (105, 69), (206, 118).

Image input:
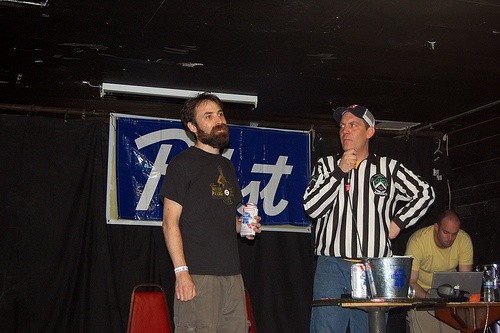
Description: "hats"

(333, 104), (375, 127)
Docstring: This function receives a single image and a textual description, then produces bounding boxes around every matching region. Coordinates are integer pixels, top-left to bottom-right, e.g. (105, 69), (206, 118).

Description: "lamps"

(100, 82), (258, 109)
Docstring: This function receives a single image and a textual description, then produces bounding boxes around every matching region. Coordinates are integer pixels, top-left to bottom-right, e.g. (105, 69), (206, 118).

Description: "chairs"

(128, 284), (170, 333)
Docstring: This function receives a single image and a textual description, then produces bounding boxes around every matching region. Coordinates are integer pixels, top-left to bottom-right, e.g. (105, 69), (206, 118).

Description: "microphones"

(346, 170), (351, 192)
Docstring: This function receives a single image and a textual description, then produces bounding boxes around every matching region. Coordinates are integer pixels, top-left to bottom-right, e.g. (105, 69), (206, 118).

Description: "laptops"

(430, 272), (483, 298)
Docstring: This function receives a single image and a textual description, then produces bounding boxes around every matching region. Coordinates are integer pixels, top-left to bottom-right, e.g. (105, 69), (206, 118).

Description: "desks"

(311, 298), (500, 333)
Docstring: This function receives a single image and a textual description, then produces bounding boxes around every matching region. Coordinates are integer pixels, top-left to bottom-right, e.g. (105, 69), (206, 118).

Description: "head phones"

(437, 284), (469, 301)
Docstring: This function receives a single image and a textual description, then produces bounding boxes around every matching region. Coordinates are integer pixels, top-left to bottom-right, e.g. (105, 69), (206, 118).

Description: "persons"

(404, 209), (473, 333)
(302, 105), (435, 333)
(160, 94), (263, 333)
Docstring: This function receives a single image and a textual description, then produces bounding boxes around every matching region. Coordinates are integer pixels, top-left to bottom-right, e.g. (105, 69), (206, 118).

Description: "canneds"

(240, 203), (258, 237)
(365, 262), (377, 296)
(350, 263), (367, 299)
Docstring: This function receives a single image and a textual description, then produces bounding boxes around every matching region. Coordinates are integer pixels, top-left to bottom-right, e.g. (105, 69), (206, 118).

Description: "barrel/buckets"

(365, 256), (415, 303)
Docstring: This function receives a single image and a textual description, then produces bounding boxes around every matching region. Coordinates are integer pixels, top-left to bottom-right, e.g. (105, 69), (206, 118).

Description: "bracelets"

(174, 266), (188, 273)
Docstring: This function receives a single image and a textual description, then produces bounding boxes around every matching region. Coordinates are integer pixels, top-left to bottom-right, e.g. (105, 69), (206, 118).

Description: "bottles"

(484, 271), (496, 303)
